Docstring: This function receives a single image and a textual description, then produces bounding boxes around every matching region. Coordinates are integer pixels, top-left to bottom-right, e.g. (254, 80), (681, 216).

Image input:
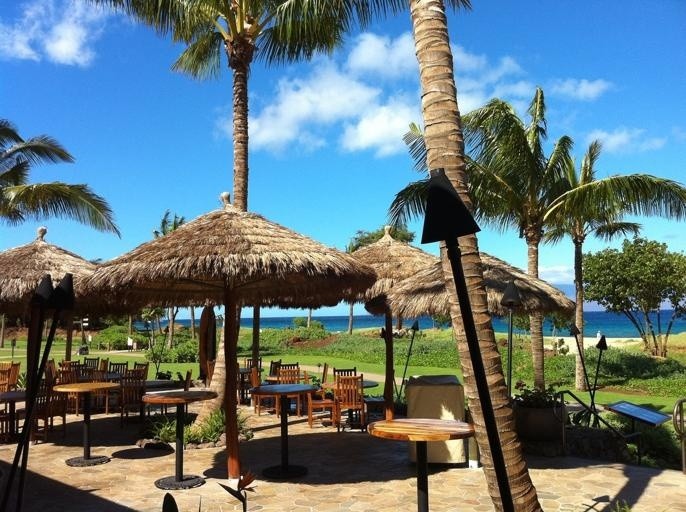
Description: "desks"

(0, 391), (27, 441)
(50, 381), (120, 468)
(140, 389), (216, 489)
(366, 416), (474, 512)
(251, 381), (320, 480)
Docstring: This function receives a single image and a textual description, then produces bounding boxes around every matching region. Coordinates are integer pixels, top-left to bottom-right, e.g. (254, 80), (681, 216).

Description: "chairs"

(0, 361), (20, 444)
(30, 357), (149, 442)
(238, 358), (368, 434)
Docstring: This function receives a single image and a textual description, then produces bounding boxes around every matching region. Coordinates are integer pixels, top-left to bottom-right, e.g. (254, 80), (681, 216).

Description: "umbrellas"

(346, 225), (440, 418)
(71, 192), (376, 478)
(383, 252), (578, 320)
(0, 227), (138, 442)
